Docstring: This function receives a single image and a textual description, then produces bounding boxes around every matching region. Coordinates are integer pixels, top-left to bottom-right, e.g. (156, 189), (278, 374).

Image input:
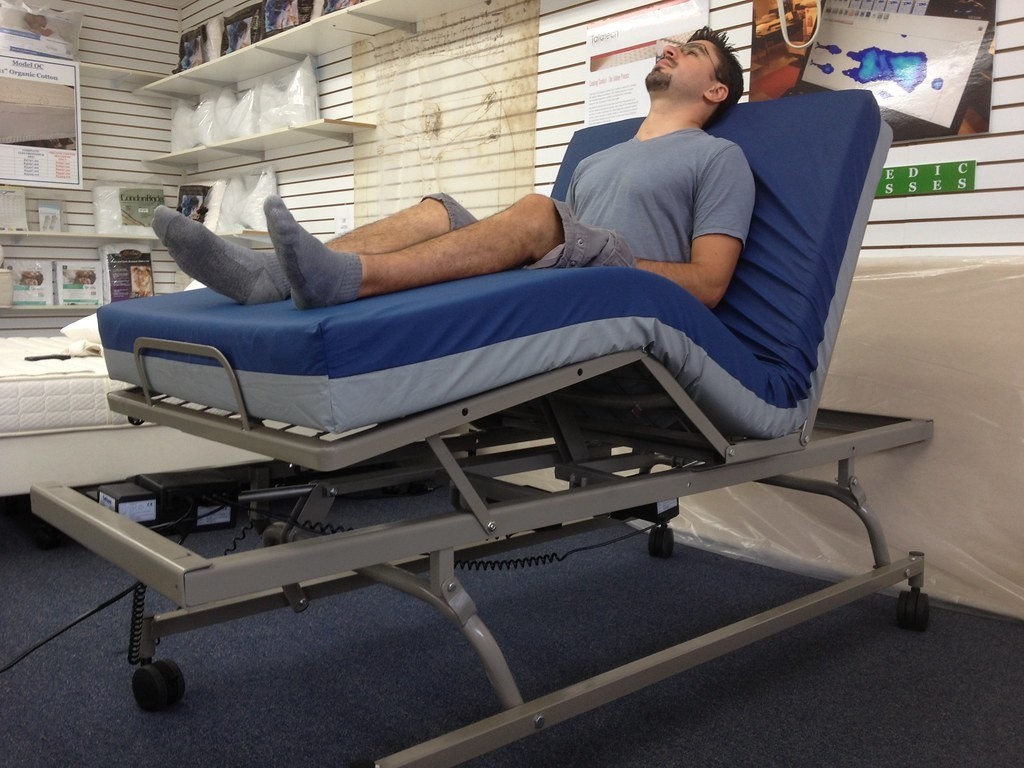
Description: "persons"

(151, 24), (755, 311)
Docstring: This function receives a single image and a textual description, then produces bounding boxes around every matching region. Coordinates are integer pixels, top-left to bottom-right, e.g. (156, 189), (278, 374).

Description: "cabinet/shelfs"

(0, 0), (473, 310)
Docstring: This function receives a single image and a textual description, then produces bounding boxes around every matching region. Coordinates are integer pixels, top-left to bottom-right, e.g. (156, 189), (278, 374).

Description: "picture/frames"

(170, 25), (206, 74)
(220, 1), (263, 57)
(262, 0), (312, 39)
(321, 0), (364, 16)
(176, 185), (209, 222)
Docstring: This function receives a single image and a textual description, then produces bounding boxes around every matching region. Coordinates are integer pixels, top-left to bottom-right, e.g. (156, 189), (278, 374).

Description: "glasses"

(678, 43), (724, 85)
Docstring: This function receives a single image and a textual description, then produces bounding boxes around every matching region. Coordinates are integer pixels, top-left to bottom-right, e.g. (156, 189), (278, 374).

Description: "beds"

(29, 90), (934, 768)
(0, 333), (458, 496)
